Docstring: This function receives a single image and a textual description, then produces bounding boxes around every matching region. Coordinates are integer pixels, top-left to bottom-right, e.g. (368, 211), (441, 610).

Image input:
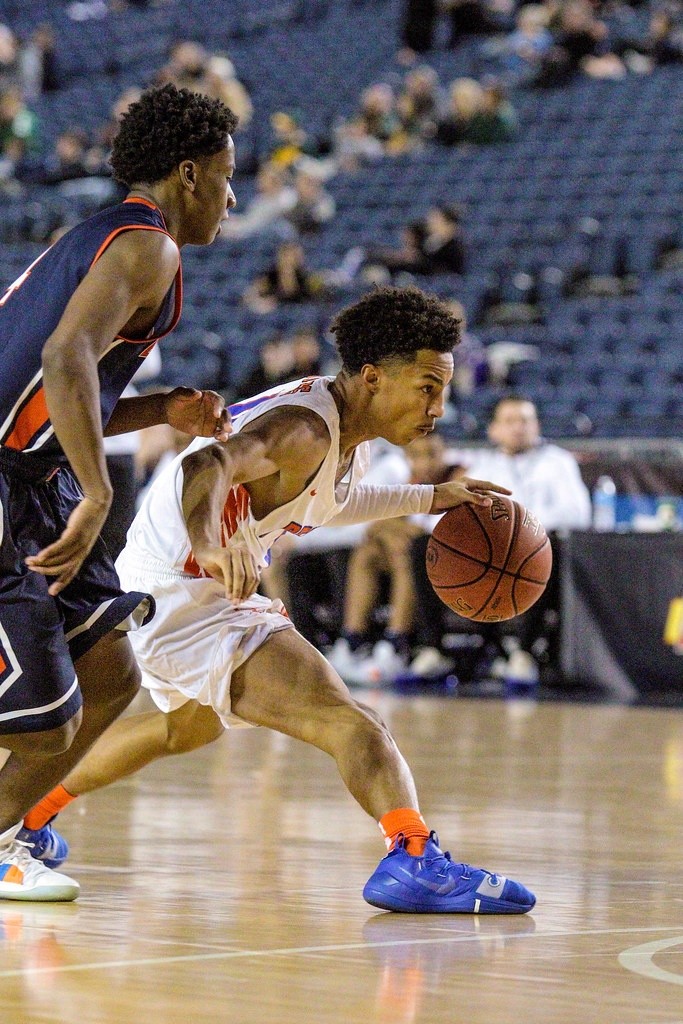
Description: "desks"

(260, 526), (683, 709)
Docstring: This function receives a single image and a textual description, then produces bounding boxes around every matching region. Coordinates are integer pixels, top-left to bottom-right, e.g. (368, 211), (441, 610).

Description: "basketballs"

(423, 494), (553, 624)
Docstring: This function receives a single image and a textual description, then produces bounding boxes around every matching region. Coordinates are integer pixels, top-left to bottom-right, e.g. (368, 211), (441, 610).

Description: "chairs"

(0, 0), (683, 438)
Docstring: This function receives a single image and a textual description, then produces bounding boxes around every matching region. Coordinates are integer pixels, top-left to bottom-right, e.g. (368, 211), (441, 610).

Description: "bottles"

(593, 475), (616, 533)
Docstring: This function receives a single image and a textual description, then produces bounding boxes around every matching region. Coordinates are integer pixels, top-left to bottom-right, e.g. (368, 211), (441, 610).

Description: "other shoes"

(350, 640), (408, 685)
(326, 638), (369, 682)
(507, 650), (540, 684)
(410, 648), (453, 674)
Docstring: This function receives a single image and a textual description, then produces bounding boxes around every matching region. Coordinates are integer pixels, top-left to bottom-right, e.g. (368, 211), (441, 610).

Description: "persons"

(2, 81), (236, 899)
(3, 0), (683, 239)
(392, 394), (595, 692)
(237, 197), (497, 404)
(261, 437), (410, 656)
(94, 340), (161, 560)
(15, 283), (539, 918)
(319, 419), (479, 688)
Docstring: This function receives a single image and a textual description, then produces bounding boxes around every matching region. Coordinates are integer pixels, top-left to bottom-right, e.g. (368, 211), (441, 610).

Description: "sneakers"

(15, 813), (70, 869)
(363, 831), (537, 913)
(0, 819), (80, 901)
